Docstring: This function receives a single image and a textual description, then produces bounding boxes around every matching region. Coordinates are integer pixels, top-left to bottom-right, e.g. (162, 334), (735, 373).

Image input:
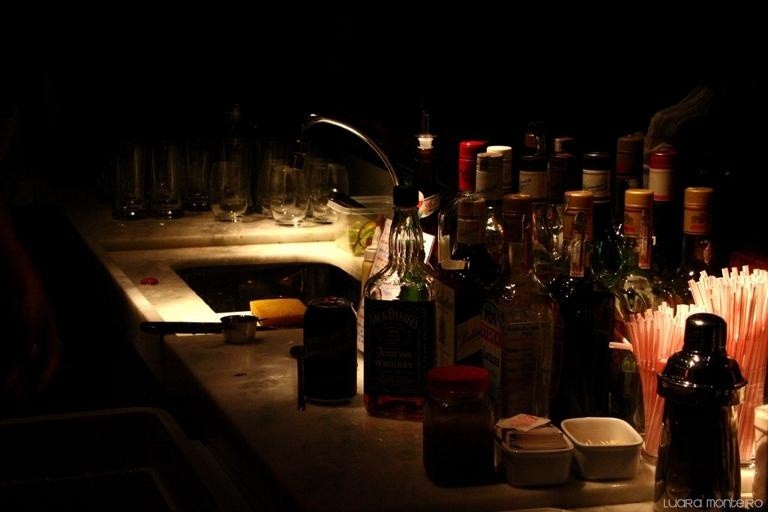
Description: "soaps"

(249, 297), (308, 328)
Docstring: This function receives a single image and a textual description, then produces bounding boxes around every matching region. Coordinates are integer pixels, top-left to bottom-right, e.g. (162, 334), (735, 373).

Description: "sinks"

(164, 259), (363, 313)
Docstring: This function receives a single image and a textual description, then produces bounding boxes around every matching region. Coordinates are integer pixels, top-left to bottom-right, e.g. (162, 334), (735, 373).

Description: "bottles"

(358, 130), (719, 430)
(296, 297), (358, 406)
(652, 312), (744, 510)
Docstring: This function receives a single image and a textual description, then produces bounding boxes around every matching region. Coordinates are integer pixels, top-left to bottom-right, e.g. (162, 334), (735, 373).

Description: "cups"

(214, 157), (339, 225)
(107, 133), (214, 219)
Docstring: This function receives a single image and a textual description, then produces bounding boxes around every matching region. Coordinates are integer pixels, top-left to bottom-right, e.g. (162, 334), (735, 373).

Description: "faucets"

(292, 114), (401, 189)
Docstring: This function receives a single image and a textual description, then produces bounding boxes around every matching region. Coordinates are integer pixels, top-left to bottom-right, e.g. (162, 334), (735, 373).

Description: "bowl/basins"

(221, 314), (257, 343)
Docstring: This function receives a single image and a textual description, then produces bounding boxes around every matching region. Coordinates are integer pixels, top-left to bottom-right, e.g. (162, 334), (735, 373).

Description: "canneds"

(303, 296), (357, 403)
(423, 364), (496, 488)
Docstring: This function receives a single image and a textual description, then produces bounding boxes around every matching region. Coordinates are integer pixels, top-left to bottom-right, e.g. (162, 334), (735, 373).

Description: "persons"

(1, 217), (64, 411)
